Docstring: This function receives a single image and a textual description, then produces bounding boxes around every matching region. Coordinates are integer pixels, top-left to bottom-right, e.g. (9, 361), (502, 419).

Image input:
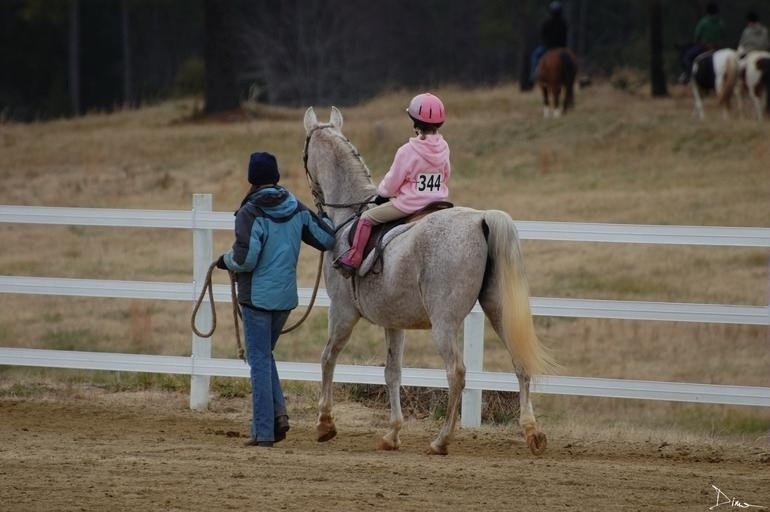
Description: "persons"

(215, 150), (338, 449)
(735, 10), (770, 53)
(681, 2), (730, 75)
(332, 91), (452, 271)
(527, 1), (567, 80)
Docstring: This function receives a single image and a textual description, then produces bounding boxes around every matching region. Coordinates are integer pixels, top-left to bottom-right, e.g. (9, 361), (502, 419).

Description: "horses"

(675, 43), (745, 120)
(534, 47), (578, 118)
(302, 104), (561, 458)
(742, 50), (770, 120)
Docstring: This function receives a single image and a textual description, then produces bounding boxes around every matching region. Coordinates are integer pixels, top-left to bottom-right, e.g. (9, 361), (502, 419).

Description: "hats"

(248, 152), (280, 184)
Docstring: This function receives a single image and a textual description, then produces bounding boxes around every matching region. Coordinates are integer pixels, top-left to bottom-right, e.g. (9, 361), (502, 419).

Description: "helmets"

(405, 93), (445, 128)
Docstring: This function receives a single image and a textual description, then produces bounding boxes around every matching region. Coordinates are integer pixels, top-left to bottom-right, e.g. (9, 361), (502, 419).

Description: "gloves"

(375, 195), (389, 204)
(217, 255), (229, 270)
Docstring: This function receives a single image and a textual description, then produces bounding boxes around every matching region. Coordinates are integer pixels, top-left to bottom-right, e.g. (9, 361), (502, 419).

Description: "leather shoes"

(243, 416), (289, 448)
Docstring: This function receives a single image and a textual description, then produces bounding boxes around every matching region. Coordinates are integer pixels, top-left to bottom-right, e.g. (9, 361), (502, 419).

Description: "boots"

(336, 219), (373, 271)
